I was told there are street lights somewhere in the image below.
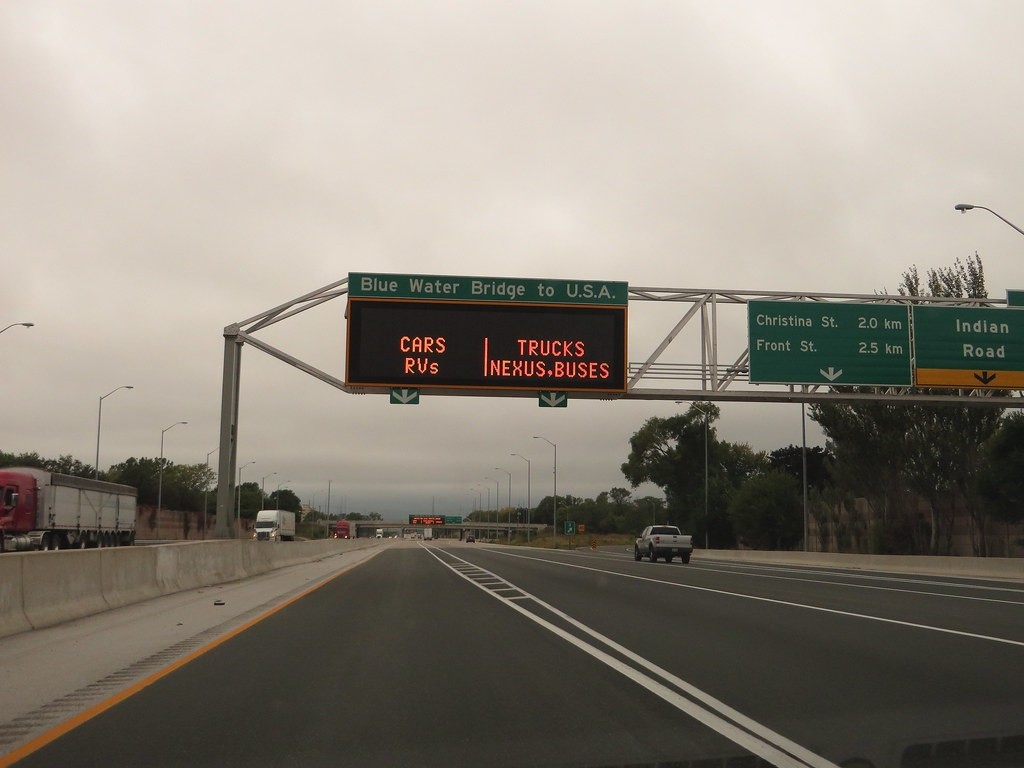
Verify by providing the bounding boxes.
[276,480,291,509]
[156,421,189,540]
[237,461,256,536]
[311,489,326,540]
[534,436,557,551]
[484,476,499,539]
[674,401,709,550]
[94,385,134,482]
[464,482,490,542]
[494,467,511,545]
[511,453,532,543]
[261,472,277,510]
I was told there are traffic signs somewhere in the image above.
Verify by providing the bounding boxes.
[911,303,1024,392]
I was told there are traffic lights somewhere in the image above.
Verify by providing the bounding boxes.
[746,298,914,389]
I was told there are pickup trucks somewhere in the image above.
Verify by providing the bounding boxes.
[634,524,693,565]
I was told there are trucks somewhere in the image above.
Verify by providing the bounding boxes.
[333,520,350,539]
[424,529,432,541]
[375,528,383,539]
[252,509,296,542]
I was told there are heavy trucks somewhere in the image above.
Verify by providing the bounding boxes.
[0,466,140,553]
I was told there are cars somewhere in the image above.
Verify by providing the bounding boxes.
[466,535,476,543]
